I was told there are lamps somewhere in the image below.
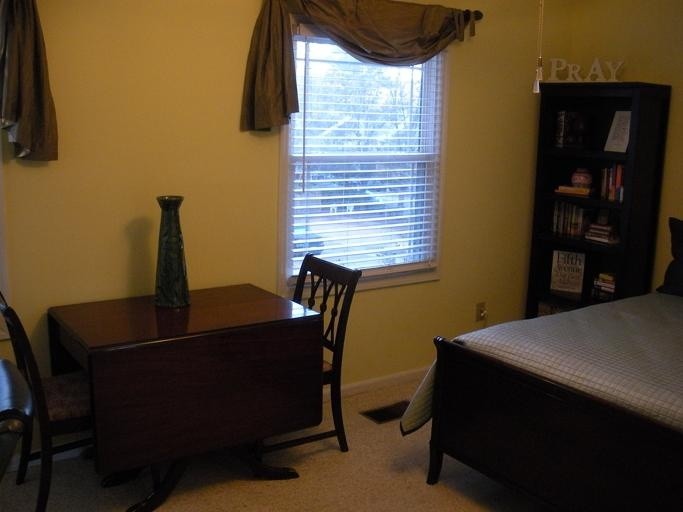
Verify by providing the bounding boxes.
[532,0,546,94]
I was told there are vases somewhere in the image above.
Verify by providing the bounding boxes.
[151,196,189,310]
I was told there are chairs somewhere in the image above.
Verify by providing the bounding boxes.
[1,359,34,479]
[265,253,362,454]
[1,293,93,512]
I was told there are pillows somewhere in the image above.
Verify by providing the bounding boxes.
[656,216,683,295]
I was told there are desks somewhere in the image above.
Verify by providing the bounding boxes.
[49,285,323,512]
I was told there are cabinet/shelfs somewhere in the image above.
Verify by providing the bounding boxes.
[524,81,670,319]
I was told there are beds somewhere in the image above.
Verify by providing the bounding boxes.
[399,293,683,512]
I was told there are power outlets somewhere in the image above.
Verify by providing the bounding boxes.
[476,302,487,320]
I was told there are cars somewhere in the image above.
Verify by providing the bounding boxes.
[288,220,324,262]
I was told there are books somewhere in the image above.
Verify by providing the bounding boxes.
[536,162,624,315]
[552,111,587,150]
[601,109,633,153]
